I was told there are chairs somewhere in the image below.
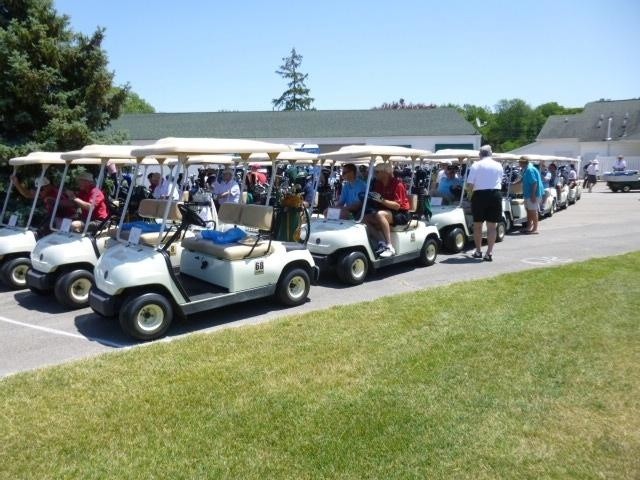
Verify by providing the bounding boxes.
[297,191,319,222]
[84,200,119,236]
[181,202,274,261]
[178,190,189,205]
[393,193,419,231]
[507,181,527,219]
[108,198,184,247]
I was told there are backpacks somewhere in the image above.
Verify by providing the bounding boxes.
[419,189,432,219]
[267,194,311,241]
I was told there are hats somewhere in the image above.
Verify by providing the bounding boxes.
[517,155,528,162]
[78,172,94,183]
[373,163,394,174]
[33,175,54,188]
[222,168,234,175]
[592,160,598,164]
[480,144,492,156]
[617,155,624,158]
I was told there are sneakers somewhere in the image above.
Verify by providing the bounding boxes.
[483,254,493,261]
[526,230,542,234]
[520,229,532,233]
[472,252,483,258]
[374,244,386,255]
[379,248,396,258]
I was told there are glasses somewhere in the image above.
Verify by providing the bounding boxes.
[343,171,349,175]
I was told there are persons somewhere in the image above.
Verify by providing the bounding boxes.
[2,156,585,234]
[366,163,410,258]
[583,161,592,189]
[612,155,626,172]
[586,160,599,193]
[467,144,504,261]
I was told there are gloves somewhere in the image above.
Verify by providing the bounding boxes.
[371,192,384,204]
